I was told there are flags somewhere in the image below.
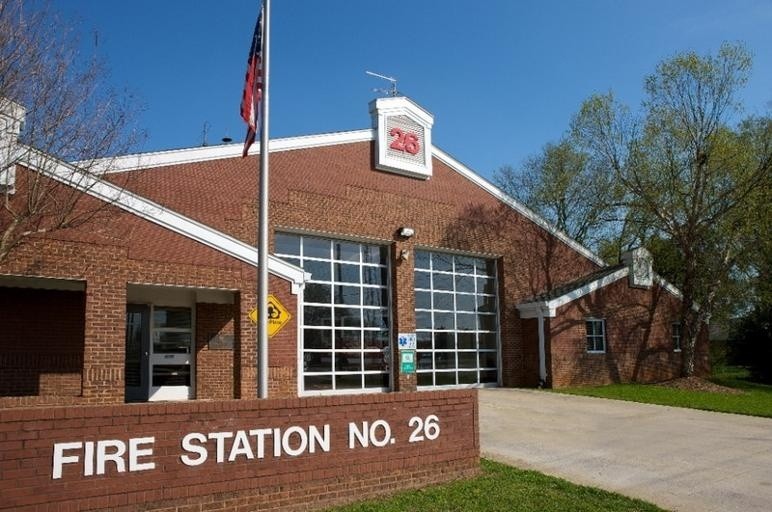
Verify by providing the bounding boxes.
[241,1,266,157]
